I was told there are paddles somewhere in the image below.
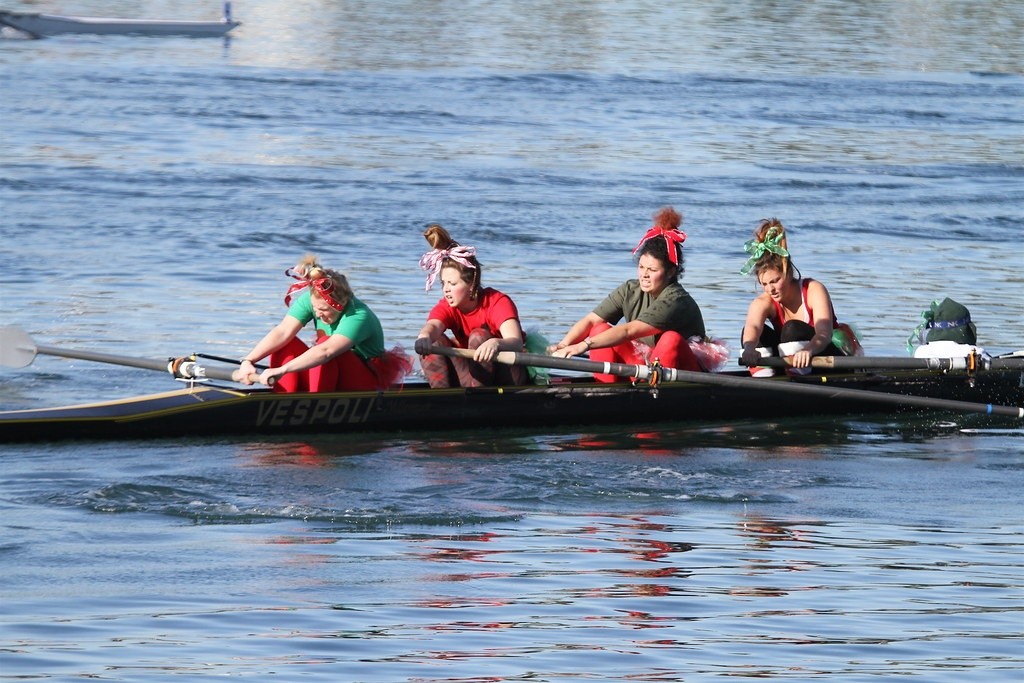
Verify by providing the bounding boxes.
[430,346,1024,418]
[735,353,1024,374]
[0,327,277,387]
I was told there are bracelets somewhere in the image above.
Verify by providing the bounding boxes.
[241,359,254,363]
[585,338,593,350]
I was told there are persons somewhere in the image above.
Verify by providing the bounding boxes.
[550,208,731,385]
[911,296,994,361]
[741,217,863,377]
[238,254,414,393]
[415,225,554,390]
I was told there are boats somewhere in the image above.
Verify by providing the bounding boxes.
[0,350,1024,437]
